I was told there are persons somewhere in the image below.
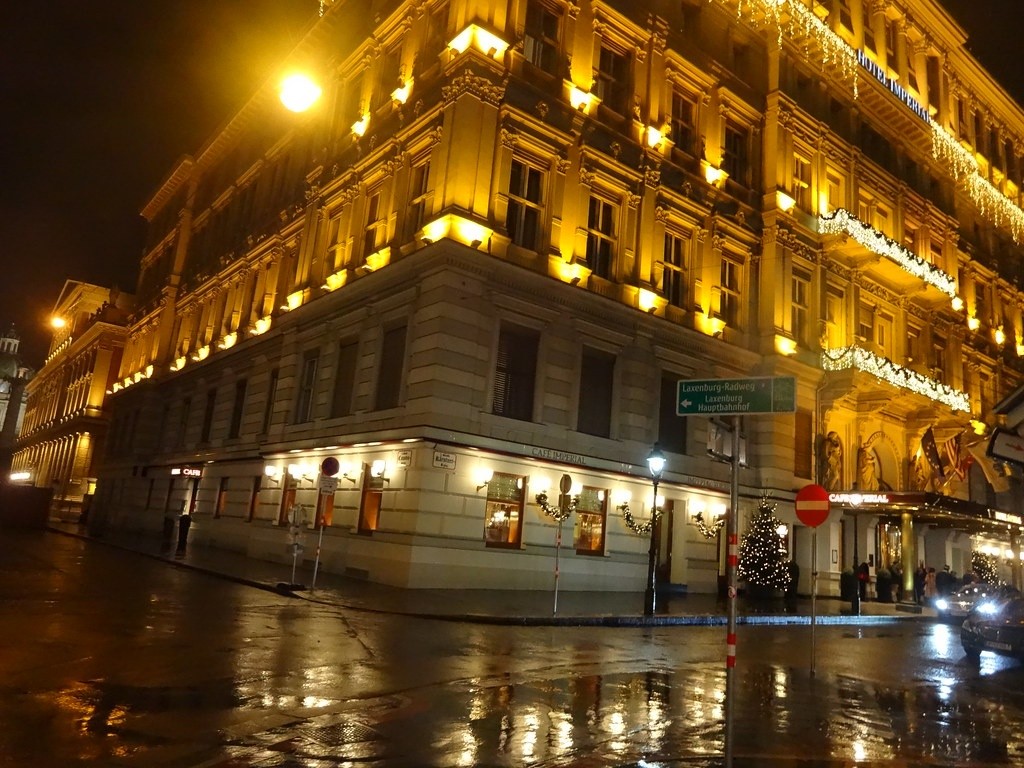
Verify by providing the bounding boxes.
[913,564,973,605]
[821,431,842,492]
[909,455,924,492]
[857,442,879,491]
[840,558,903,604]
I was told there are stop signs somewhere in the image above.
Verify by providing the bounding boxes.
[795,484,830,527]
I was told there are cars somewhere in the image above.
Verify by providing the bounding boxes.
[938,584,993,619]
[960,595,1024,658]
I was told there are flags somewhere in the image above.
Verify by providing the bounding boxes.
[964,434,1010,492]
[943,432,966,482]
[921,428,945,477]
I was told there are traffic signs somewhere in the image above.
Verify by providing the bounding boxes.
[675,376,796,415]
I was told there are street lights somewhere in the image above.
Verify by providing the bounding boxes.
[847,482,863,639]
[644,442,666,616]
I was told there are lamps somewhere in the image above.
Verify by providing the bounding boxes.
[450,47,460,57]
[340,461,356,484]
[652,142,662,152]
[711,179,720,187]
[393,99,402,106]
[785,207,794,214]
[570,277,581,285]
[713,330,722,337]
[280,306,289,312]
[487,46,497,59]
[420,238,432,245]
[470,239,482,249]
[265,465,279,484]
[578,102,587,112]
[371,460,389,484]
[362,264,372,274]
[648,306,657,314]
